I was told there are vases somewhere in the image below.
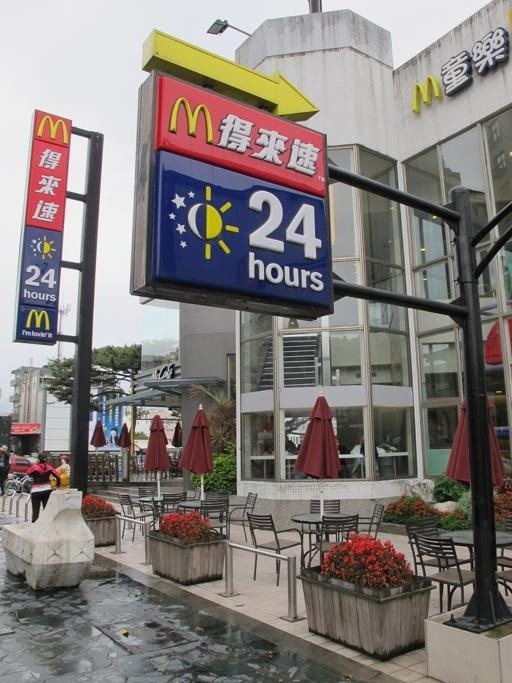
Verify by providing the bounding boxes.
[83,516,117,547]
[297,566,437,662]
[145,530,227,587]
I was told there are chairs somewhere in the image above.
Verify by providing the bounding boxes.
[195,487,201,500]
[342,504,385,542]
[152,497,173,531]
[201,499,229,536]
[320,514,359,566]
[118,492,154,542]
[206,491,229,508]
[496,570,512,597]
[228,492,258,546]
[138,487,160,531]
[310,500,340,515]
[246,512,303,587]
[404,517,470,577]
[163,491,187,508]
[412,531,476,611]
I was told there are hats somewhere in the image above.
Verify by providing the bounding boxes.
[59,454,70,464]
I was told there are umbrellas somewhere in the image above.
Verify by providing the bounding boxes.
[172,421,182,452]
[90,421,106,455]
[445,397,505,486]
[145,415,173,497]
[179,404,214,500]
[117,423,131,448]
[292,393,339,521]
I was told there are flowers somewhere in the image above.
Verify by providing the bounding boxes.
[319,533,415,589]
[158,510,210,542]
[82,495,121,519]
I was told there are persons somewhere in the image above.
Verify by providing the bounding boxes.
[263,435,296,464]
[49,456,70,489]
[27,453,61,523]
[350,435,386,479]
[335,437,350,479]
[0,445,10,495]
[9,450,15,456]
[31,447,41,453]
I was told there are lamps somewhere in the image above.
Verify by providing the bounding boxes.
[207,18,250,37]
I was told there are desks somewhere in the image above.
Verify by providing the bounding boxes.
[291,514,355,580]
[438,531,512,593]
